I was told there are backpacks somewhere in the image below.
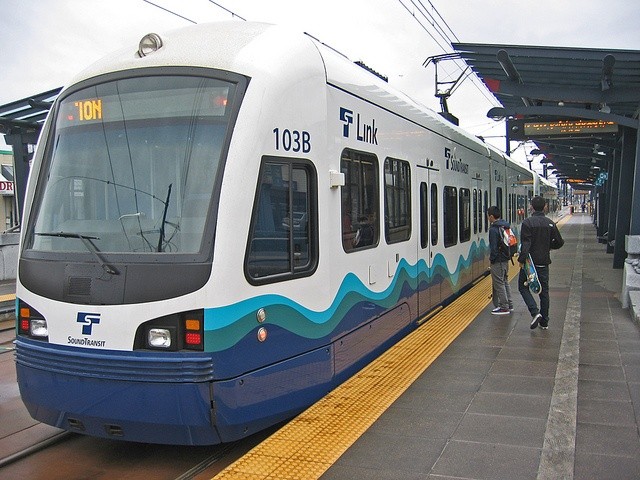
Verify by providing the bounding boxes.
[497,225,517,260]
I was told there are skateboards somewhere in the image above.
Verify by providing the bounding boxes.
[514,243,542,295]
[488,259,497,308]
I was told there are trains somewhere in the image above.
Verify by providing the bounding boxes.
[13,21,563,449]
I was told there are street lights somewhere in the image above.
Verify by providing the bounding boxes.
[529,147,607,160]
[486,104,639,131]
[547,165,598,184]
[539,157,600,167]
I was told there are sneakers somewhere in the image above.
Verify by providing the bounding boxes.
[530,313,543,330]
[491,306,510,315]
[509,306,514,312]
[538,323,548,330]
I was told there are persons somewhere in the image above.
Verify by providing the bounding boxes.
[487,206,517,315]
[519,196,564,328]
